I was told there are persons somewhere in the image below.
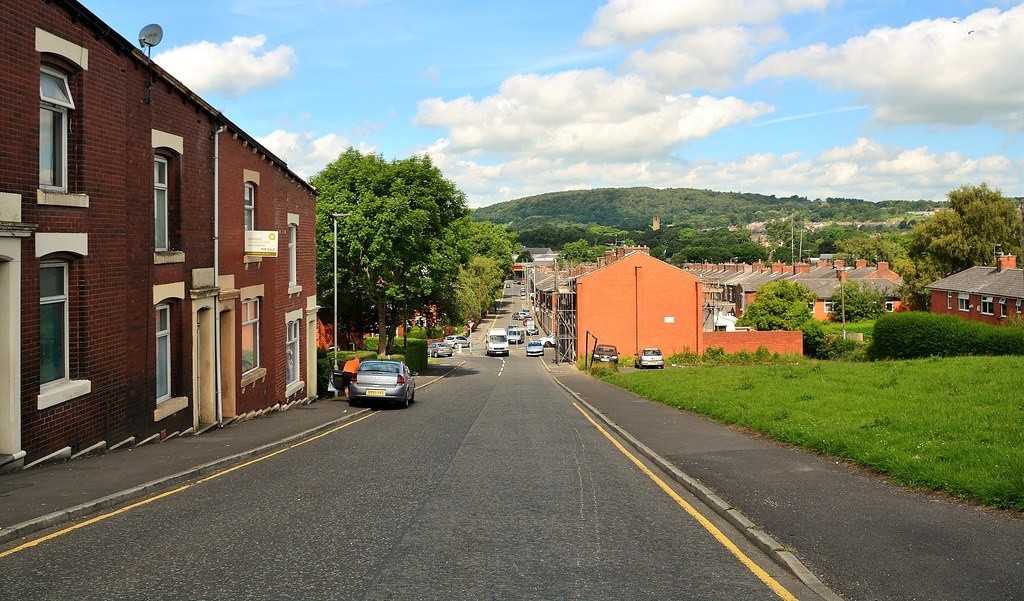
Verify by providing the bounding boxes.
[342,355,361,403]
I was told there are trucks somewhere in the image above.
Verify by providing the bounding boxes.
[485,328,510,357]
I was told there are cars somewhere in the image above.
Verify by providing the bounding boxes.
[427,341,453,357]
[443,335,471,349]
[524,341,545,357]
[591,344,620,364]
[327,358,419,408]
[539,333,556,347]
[634,348,665,369]
[506,280,539,343]
